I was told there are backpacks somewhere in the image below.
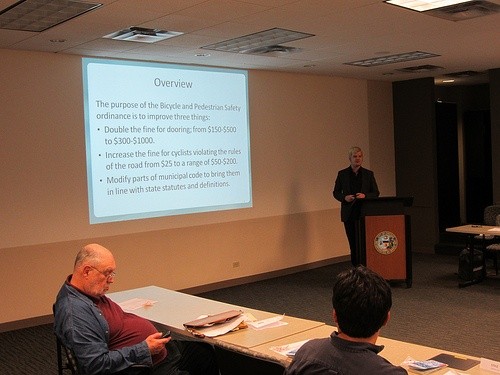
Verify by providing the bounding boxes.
[457,247,486,281]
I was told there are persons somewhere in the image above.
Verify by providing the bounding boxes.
[283,265,409,375]
[53,243,220,375]
[333,147,380,269]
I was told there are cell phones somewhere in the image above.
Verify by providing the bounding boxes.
[162,331,171,338]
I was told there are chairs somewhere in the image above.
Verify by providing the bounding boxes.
[486,214,500,274]
[53,302,85,375]
[467,204,500,241]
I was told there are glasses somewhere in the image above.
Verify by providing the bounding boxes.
[91,266,117,280]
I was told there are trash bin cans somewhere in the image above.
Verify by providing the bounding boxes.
[459,247,484,284]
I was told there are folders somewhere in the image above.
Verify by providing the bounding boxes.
[183,309,244,337]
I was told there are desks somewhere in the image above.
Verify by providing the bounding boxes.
[104,285,500,375]
[448,223,500,278]
